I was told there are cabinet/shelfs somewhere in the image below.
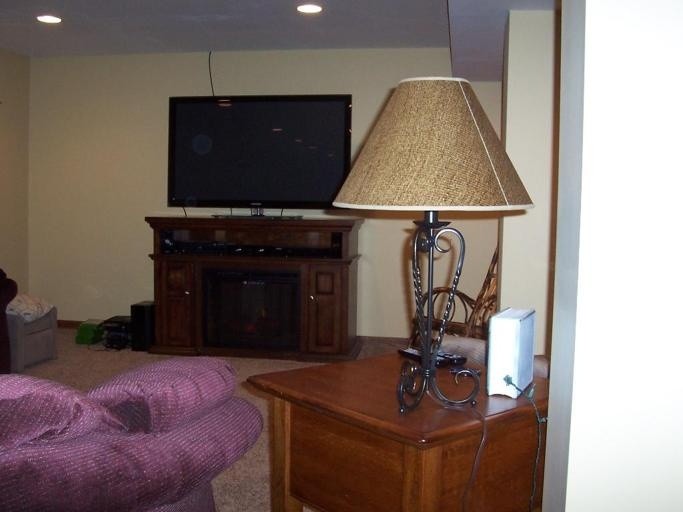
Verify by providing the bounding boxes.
[145,217,365,361]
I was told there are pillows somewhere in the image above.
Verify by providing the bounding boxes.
[0,373,130,454]
[84,357,238,436]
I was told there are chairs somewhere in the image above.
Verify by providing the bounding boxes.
[408,243,498,350]
[5,305,58,372]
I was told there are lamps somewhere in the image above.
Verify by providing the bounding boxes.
[331,76,534,416]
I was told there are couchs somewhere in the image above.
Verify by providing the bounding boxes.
[0,355,263,512]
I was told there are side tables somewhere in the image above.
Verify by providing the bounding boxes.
[247,350,549,512]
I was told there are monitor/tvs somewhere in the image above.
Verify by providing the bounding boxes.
[167,94,352,220]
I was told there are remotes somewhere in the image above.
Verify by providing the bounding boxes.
[398,347,450,367]
[410,344,467,366]
